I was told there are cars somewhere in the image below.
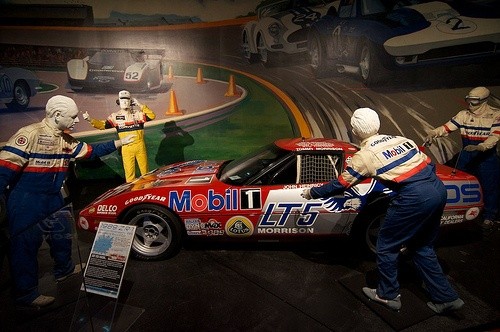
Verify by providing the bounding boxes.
[77,138,484,263]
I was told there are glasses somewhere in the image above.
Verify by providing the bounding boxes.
[465,96,489,105]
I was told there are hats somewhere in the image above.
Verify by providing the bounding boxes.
[118,91,131,99]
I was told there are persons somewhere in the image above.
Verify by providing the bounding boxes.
[301,107,464,313]
[0,95,136,305]
[82,90,156,182]
[427,86,500,237]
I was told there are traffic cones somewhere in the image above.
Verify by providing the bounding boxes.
[165,91,184,116]
[223,74,243,98]
[195,66,207,84]
[166,66,176,80]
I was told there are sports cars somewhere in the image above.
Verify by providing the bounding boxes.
[241,0,340,68]
[306,0,500,87]
[64,47,166,93]
[0,66,41,111]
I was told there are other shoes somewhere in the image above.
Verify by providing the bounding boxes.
[480,220,494,228]
[32,295,55,307]
[427,298,465,314]
[363,287,400,309]
[57,262,87,280]
